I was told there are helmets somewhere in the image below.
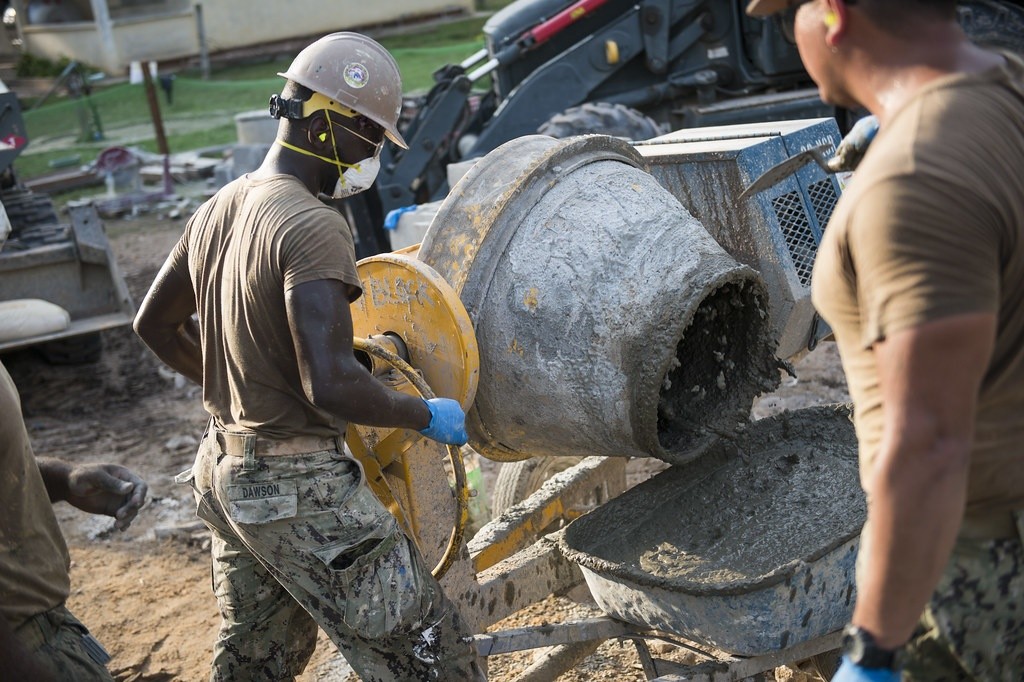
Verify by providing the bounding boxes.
[277,31,410,152]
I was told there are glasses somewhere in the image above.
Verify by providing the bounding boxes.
[320,115,387,160]
[774,0,859,47]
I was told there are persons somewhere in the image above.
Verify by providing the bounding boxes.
[132,31,486,681]
[0,358,149,682]
[744,0,1024,682]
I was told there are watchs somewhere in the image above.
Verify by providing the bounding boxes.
[841,623,905,668]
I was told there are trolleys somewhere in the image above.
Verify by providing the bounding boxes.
[462,400,870,681]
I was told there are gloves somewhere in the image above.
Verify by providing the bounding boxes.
[383,202,418,228]
[419,397,468,447]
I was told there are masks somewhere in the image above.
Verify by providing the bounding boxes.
[332,138,382,199]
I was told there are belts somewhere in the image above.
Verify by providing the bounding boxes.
[956,503,1024,542]
[205,417,337,466]
